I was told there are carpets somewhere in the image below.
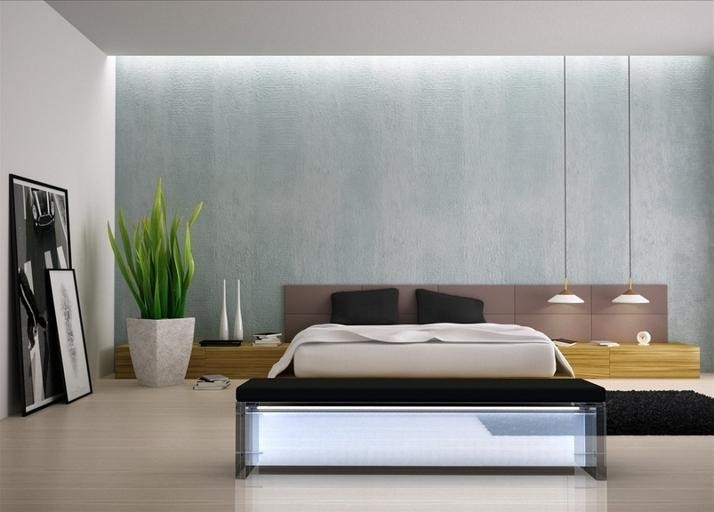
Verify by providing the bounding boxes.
[602,389,713,438]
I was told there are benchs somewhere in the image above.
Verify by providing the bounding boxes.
[231,377,611,483]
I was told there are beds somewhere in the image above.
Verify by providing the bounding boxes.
[289,320,560,378]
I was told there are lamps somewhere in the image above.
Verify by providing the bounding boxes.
[546,56,652,305]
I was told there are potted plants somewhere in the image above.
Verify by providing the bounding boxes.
[107,172,207,387]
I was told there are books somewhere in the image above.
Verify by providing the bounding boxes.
[604,343,619,347]
[590,339,617,345]
[252,332,284,348]
[551,337,576,346]
[192,374,231,391]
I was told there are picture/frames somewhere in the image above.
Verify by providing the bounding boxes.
[10,172,94,417]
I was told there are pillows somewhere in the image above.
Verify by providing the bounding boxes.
[329,287,487,324]
